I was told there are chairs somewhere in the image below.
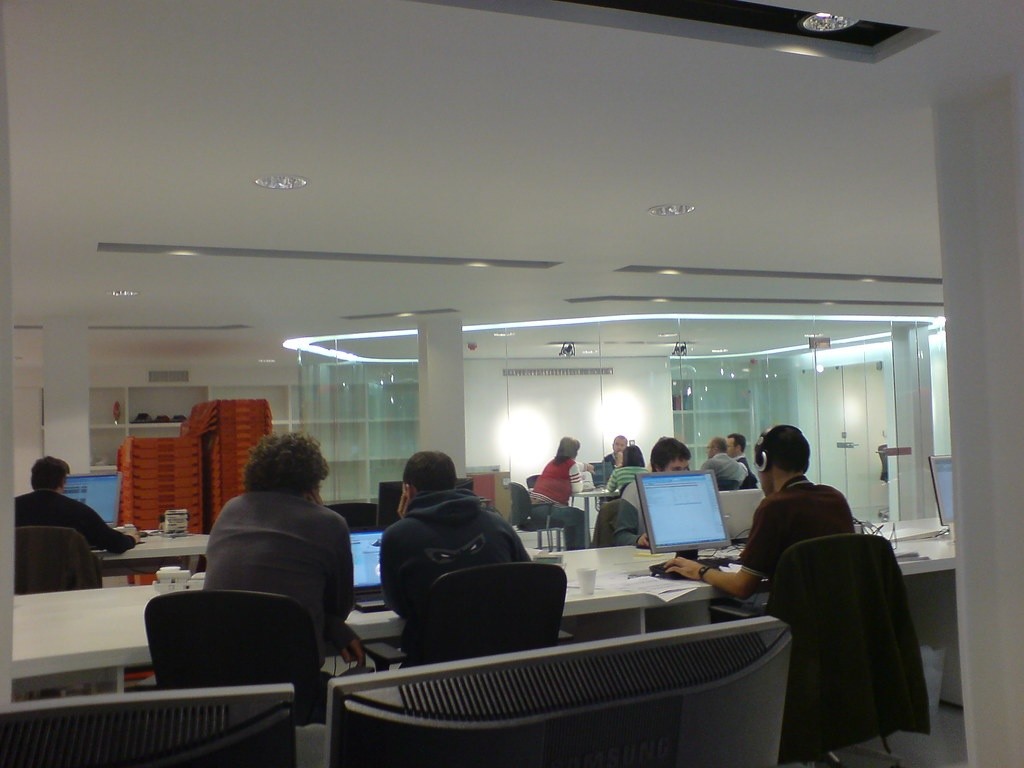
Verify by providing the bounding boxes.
[510,482,566,554]
[15,526,102,595]
[145,593,319,727]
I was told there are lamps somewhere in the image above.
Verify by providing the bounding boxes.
[671,341,687,356]
[559,341,575,357]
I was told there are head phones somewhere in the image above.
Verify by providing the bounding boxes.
[753,424,801,472]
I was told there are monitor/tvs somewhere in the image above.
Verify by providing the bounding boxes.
[634,469,733,560]
[61,471,123,528]
[928,454,955,526]
[0,682,299,768]
[326,614,792,768]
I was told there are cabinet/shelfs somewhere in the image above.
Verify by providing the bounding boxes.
[89,383,212,467]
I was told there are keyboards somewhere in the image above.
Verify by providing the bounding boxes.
[649,557,744,580]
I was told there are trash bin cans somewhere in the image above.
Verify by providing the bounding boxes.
[918,643,947,717]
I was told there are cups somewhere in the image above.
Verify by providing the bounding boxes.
[576,568,597,595]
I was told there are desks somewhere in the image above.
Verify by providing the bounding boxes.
[0,490,961,713]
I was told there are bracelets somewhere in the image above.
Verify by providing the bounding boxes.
[699,565,721,582]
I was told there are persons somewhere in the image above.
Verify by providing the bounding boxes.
[613,436,691,547]
[700,437,748,488]
[602,436,628,469]
[528,437,595,550]
[14,456,140,554]
[379,451,532,655]
[727,433,753,489]
[203,429,367,726]
[606,445,649,496]
[664,421,855,599]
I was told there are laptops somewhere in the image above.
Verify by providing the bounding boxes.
[589,462,614,488]
[375,481,405,529]
[348,525,393,613]
